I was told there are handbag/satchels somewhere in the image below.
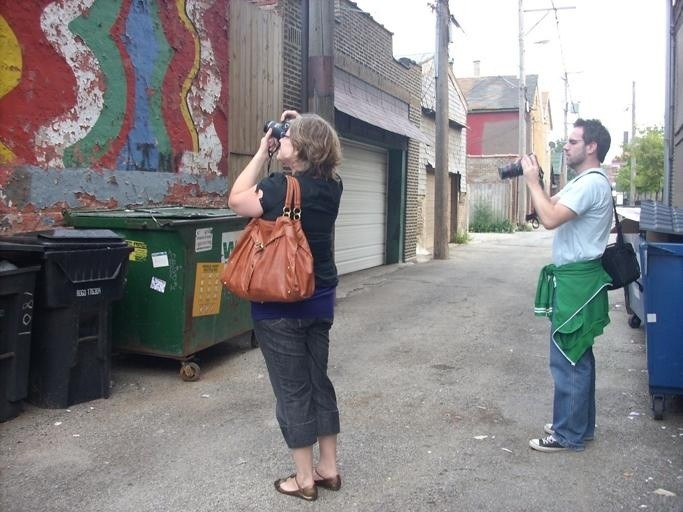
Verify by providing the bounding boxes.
[602,242,641,292]
[217,176,316,305]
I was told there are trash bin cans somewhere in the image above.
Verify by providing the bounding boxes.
[0,229,135,424]
[618,233,646,328]
[62,207,259,382]
[638,241,683,421]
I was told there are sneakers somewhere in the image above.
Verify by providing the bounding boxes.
[528,435,566,453]
[544,422,594,441]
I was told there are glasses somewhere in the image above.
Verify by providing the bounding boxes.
[280,132,289,139]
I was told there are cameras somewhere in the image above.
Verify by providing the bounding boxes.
[263,118,293,141]
[498,152,544,180]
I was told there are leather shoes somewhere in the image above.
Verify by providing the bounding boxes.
[273,475,317,501]
[288,467,341,491]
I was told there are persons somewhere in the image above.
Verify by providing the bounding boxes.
[226,106,344,499]
[514,118,615,450]
